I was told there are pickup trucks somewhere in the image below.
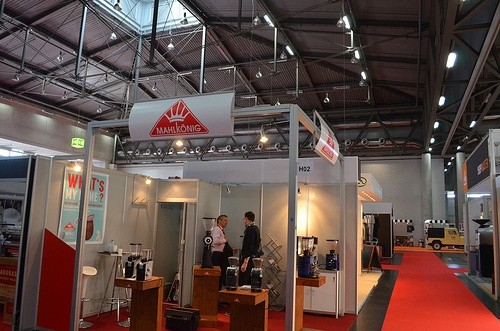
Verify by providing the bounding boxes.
[425,228,464,250]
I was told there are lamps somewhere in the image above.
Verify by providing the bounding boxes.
[180,11,189,25]
[256,66,263,79]
[62,91,69,101]
[280,45,288,60]
[337,3,368,87]
[113,0,121,9]
[56,51,64,63]
[167,38,175,49]
[226,187,231,194]
[259,124,272,143]
[152,81,159,91]
[323,91,330,103]
[252,11,263,25]
[109,18,117,38]
[97,105,103,112]
[12,74,20,82]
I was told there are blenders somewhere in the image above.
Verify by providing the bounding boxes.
[225,257,239,291]
[297,238,318,278]
[249,258,263,292]
[325,239,339,271]
[124,242,153,282]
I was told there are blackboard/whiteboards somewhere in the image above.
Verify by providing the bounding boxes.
[363,245,380,268]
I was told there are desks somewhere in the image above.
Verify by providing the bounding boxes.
[191,264,221,322]
[295,276,326,330]
[217,285,269,331]
[114,277,163,331]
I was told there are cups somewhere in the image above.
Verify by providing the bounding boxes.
[112,244,117,252]
[118,249,123,254]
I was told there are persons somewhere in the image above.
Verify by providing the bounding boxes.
[238,212,261,286]
[211,215,229,266]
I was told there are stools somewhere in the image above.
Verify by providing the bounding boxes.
[78,265,97,329]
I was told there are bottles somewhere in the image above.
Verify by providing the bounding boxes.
[109,240,115,252]
